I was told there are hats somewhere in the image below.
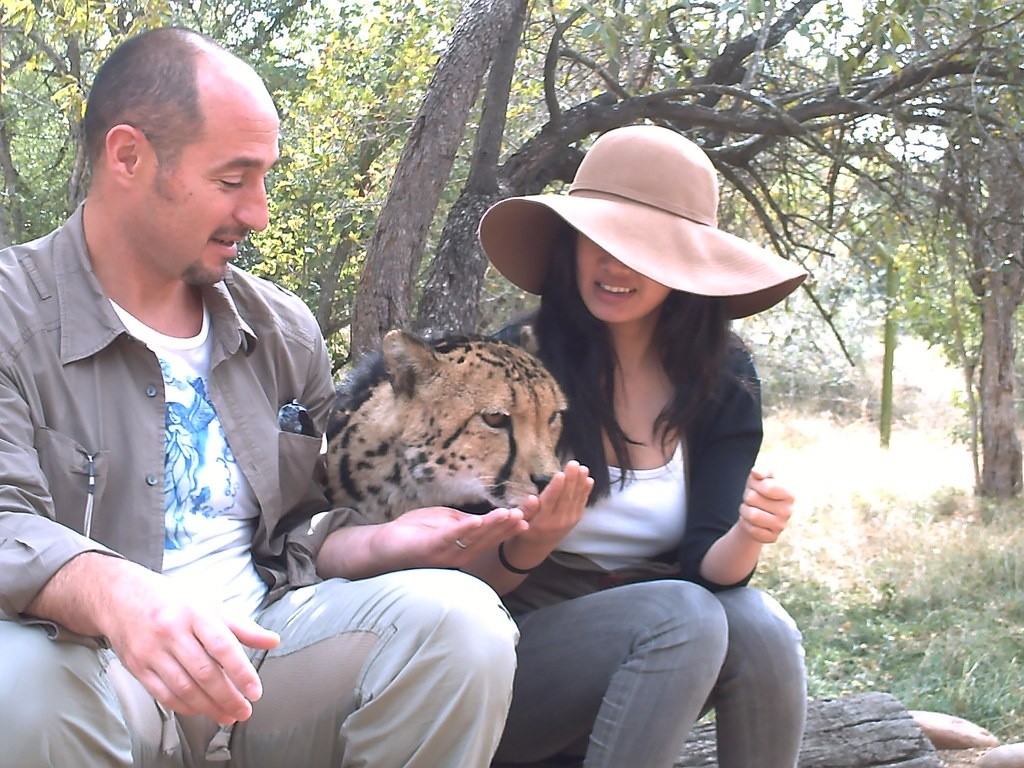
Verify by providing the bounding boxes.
[477,125,808,320]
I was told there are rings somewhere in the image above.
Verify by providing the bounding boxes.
[455,539,467,549]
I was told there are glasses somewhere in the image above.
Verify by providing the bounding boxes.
[278,398,315,437]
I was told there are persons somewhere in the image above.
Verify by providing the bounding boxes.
[478,126,810,768]
[0,27,530,768]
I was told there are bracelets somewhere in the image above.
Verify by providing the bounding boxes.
[498,541,548,574]
